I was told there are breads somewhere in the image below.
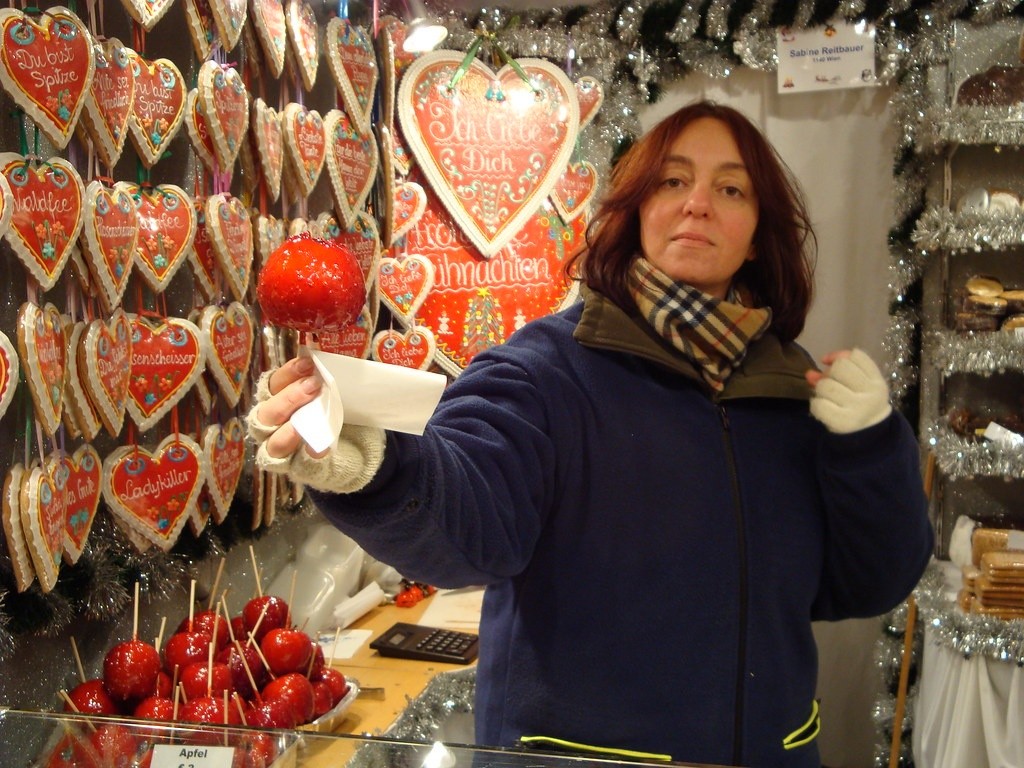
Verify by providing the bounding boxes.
[971,528,1024,567]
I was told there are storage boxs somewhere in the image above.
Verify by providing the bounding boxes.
[0,706,724,768]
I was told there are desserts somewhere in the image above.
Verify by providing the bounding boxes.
[47,596,346,767]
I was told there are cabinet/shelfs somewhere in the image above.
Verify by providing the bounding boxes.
[910,25,1024,562]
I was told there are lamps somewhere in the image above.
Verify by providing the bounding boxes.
[399,0,447,54]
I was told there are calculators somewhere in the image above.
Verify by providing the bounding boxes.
[370,622,479,665]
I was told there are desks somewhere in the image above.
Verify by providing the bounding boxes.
[286,585,490,768]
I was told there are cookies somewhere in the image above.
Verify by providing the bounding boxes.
[959,550,1024,621]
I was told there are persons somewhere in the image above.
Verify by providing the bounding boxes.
[248,101,937,768]
